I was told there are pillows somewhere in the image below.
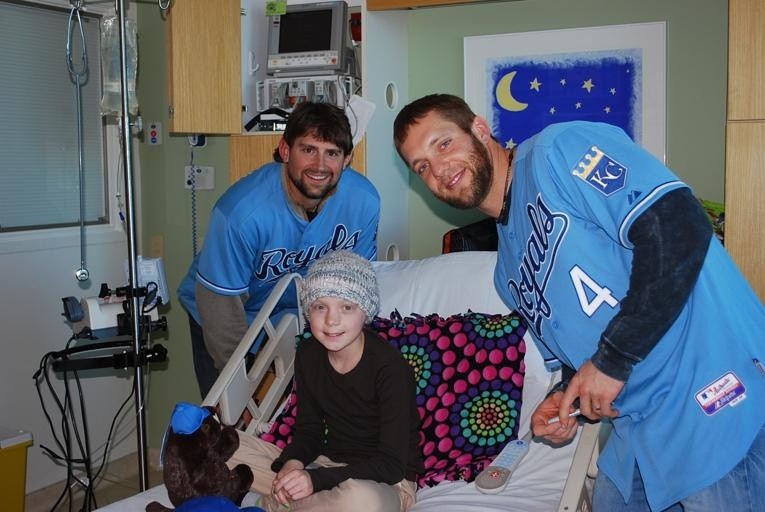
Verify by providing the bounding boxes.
[260,312,527,485]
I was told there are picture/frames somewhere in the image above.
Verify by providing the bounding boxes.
[462,21,668,172]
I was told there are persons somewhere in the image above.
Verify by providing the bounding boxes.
[240,248,421,512]
[392,93,765,512]
[176,100,381,431]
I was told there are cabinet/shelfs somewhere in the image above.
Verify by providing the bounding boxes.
[167,0,365,187]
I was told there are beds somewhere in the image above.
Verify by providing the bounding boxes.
[88,251,601,512]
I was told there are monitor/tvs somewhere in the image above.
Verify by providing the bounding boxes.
[266,1,350,74]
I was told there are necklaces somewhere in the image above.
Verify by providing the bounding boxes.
[496,151,513,224]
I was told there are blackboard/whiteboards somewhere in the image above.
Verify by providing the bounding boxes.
[0,0,110,233]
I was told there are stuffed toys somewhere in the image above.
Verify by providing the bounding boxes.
[144,401,256,511]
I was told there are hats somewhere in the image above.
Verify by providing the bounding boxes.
[298,249,382,324]
